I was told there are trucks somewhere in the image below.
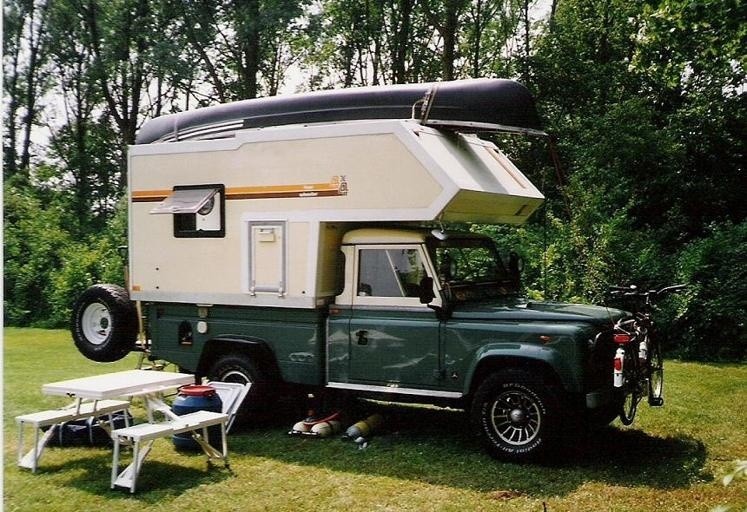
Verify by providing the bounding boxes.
[123,128,636,463]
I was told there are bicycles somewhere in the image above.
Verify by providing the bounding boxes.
[608,281,692,433]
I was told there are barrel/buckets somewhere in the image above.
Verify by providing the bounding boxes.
[172,384,222,450]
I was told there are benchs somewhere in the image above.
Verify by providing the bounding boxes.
[110,407,228,493]
[16,397,126,475]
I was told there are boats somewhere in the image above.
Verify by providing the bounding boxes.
[126,72,549,142]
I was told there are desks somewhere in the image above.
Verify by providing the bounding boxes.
[41,368,196,423]
